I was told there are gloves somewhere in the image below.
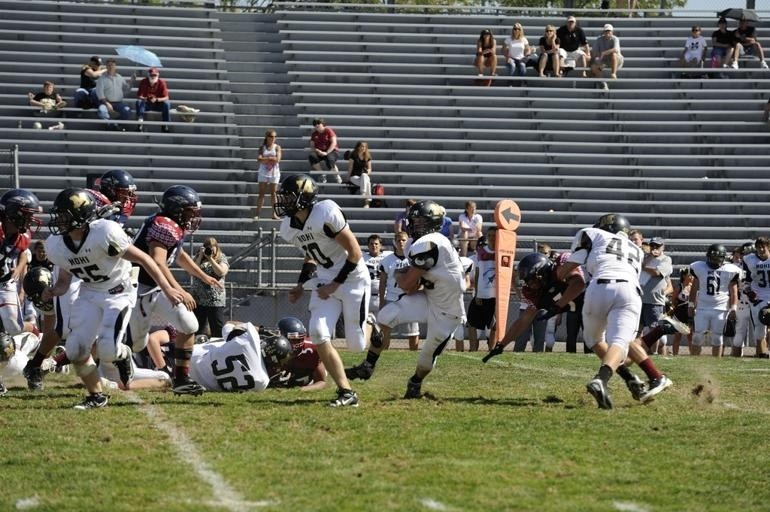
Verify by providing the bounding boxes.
[266,370,292,388]
[482,341,504,363]
[529,303,562,325]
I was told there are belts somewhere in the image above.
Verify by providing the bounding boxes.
[597,279,641,295]
[371,294,378,296]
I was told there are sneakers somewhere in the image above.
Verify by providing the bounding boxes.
[600,82,608,90]
[327,316,422,409]
[585,374,674,410]
[321,175,328,183]
[730,62,739,69]
[657,313,691,336]
[760,62,768,68]
[172,374,206,397]
[22,346,72,396]
[333,173,342,184]
[112,344,135,390]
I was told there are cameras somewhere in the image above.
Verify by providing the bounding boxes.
[203,247,214,256]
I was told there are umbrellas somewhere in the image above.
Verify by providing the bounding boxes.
[115,45,166,74]
[718,8,760,21]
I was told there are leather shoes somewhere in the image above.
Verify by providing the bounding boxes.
[105,118,171,133]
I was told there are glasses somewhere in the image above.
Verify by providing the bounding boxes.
[482,27,554,36]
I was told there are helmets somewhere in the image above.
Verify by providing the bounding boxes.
[161,185,203,234]
[0,170,138,235]
[259,317,307,368]
[405,200,446,238]
[476,235,489,252]
[22,266,53,311]
[598,214,631,239]
[273,174,319,217]
[0,331,16,362]
[517,253,556,305]
[705,244,726,269]
[760,305,770,326]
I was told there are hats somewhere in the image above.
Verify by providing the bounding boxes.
[90,56,103,65]
[567,15,577,23]
[648,236,664,246]
[603,23,613,32]
[148,68,160,76]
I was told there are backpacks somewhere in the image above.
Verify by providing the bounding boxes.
[370,182,388,208]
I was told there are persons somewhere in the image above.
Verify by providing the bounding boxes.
[0,169,498,409]
[482,213,769,408]
[135,68,173,134]
[472,15,624,90]
[308,117,343,185]
[74,56,107,118]
[679,16,768,70]
[95,58,137,132]
[347,141,373,208]
[28,80,64,131]
[253,130,282,220]
